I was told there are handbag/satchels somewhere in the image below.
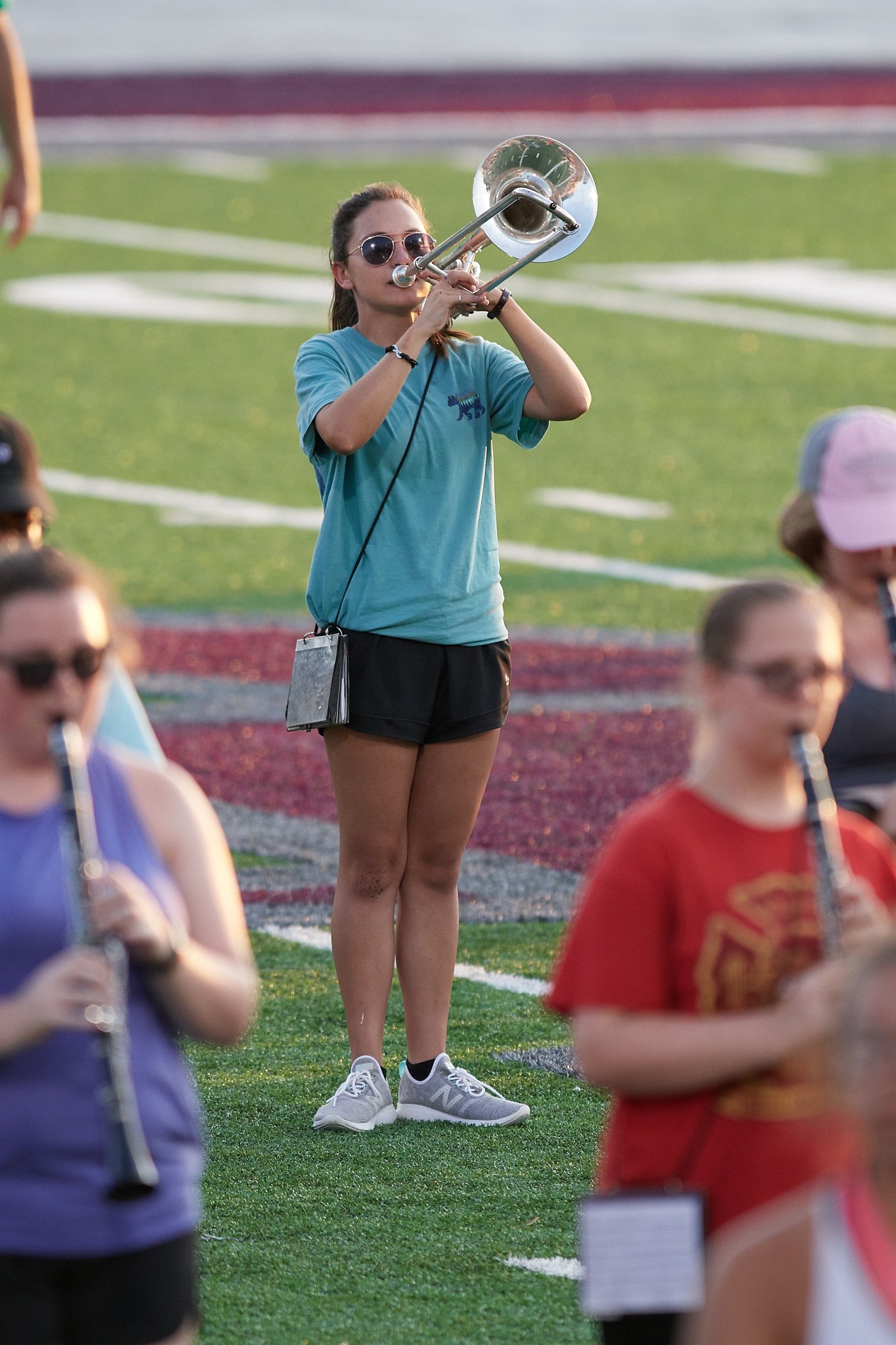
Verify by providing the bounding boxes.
[285,623,351,731]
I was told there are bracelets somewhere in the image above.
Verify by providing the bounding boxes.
[384,344,419,369]
[487,287,513,320]
[131,945,179,977]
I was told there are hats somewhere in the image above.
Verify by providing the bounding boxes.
[1,408,56,522]
[800,407,896,550]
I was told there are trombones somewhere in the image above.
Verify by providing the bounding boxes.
[392,134,599,320]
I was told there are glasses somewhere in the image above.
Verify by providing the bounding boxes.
[7,639,115,691]
[344,232,436,268]
[733,660,853,706]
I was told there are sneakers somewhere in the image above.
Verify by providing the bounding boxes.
[311,1055,396,1132]
[396,1052,531,1128]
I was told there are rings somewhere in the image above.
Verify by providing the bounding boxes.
[458,295,462,303]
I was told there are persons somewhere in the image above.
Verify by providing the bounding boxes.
[0,0,43,256]
[776,404,896,858]
[572,578,896,1342]
[1,548,259,1345]
[0,409,168,771]
[291,182,593,1129]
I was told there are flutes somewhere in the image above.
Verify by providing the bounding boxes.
[874,569,896,666]
[788,730,877,1085]
[50,712,164,1209]
